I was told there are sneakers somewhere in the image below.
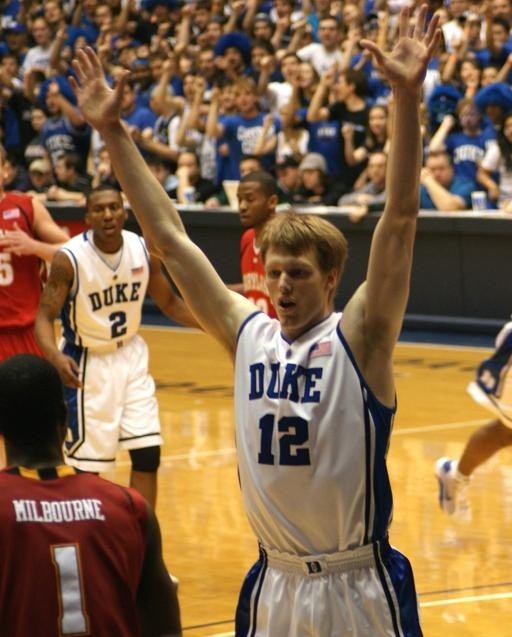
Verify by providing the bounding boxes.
[434,457,463,515]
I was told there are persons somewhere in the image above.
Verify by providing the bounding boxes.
[431,320,512,516]
[1,144,73,357]
[0,350,182,636]
[222,169,280,322]
[68,1,442,636]
[33,184,206,598]
[0,0,512,223]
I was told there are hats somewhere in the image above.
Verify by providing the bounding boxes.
[299,154,326,171]
[30,160,47,174]
